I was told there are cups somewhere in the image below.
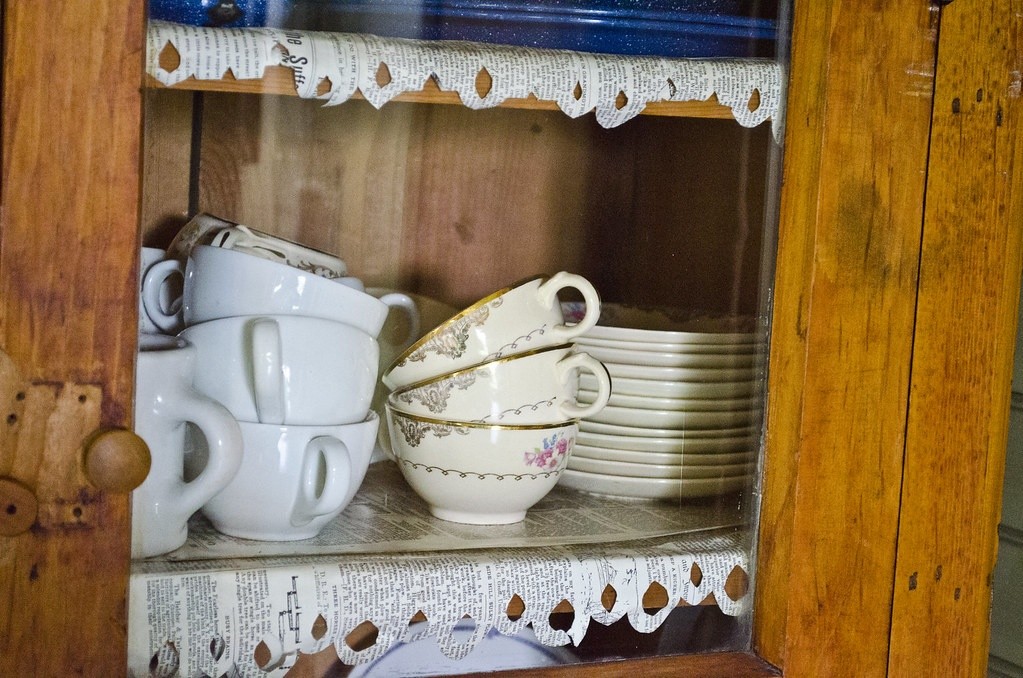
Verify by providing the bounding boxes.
[130,247,421,561]
[163,212,347,278]
[379,271,612,526]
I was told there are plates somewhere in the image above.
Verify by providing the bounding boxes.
[556,299,768,501]
[322,0,781,58]
[344,618,584,678]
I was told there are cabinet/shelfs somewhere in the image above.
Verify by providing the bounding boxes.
[0,0,1021,677]
